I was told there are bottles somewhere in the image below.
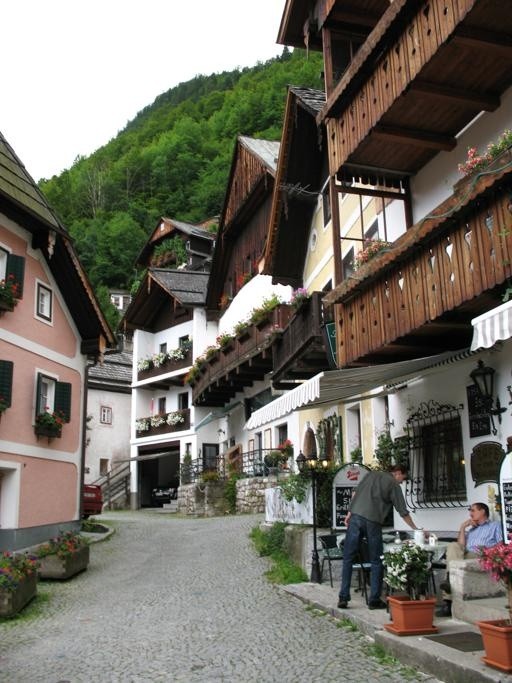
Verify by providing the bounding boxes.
[394,530,401,545]
[405,540,413,548]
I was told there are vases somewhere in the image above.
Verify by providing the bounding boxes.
[476,621,511,672]
[281,463,287,469]
[0,294,15,310]
[34,426,61,437]
[384,594,439,636]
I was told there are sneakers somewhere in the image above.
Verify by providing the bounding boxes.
[337,598,348,608]
[367,599,387,610]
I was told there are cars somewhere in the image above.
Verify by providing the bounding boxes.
[151,480,180,507]
[81,483,105,521]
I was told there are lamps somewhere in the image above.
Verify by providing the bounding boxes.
[470,358,508,434]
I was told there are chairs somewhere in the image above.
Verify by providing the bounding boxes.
[317,530,459,604]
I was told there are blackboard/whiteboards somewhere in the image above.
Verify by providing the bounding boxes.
[332,463,372,532]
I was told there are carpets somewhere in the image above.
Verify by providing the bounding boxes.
[423,630,484,652]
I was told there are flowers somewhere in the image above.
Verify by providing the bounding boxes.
[37,405,65,429]
[349,236,390,270]
[277,439,294,463]
[2,275,22,295]
[136,347,185,372]
[457,128,512,174]
[0,550,41,592]
[472,531,512,620]
[181,286,309,388]
[135,411,185,432]
[39,528,91,561]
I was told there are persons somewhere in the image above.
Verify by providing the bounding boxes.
[431,502,504,616]
[336,461,431,611]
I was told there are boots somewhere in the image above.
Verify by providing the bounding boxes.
[434,598,452,617]
[439,571,452,595]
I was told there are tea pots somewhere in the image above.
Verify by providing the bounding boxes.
[413,529,426,546]
